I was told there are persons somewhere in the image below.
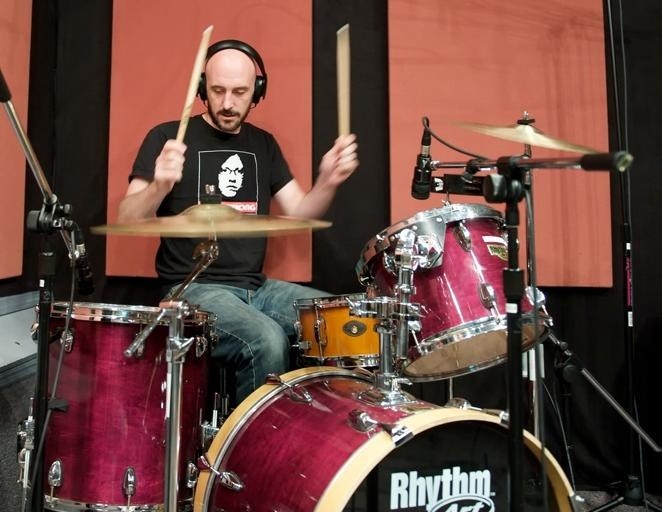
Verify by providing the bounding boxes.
[113,40,361,405]
[207,149,252,202]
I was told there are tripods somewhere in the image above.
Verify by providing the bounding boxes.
[590,1,662,511]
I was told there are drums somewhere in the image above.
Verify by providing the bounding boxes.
[16,301,218,511]
[355,203,554,381]
[292,292,379,369]
[193,365,580,512]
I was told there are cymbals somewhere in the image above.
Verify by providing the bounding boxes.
[90,205,333,238]
[437,117,599,155]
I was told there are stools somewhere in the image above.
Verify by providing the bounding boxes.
[210,357,236,417]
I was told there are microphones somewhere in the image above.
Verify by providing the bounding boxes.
[412,138,432,200]
[74,254,96,303]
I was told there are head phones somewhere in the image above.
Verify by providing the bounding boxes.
[197,40,268,105]
